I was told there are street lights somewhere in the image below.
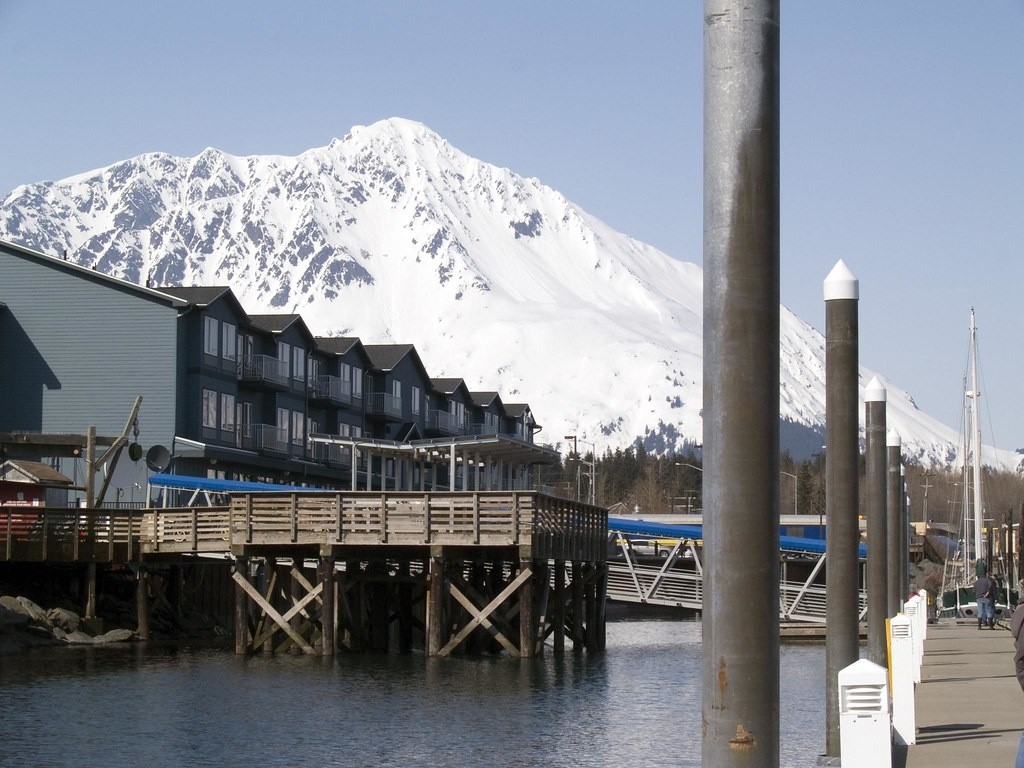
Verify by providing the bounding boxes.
[578,438,596,507]
[811,453,822,541]
[565,434,579,502]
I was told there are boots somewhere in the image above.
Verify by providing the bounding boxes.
[988,618,994,630]
[978,618,982,630]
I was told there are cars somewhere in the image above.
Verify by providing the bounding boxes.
[626,539,671,558]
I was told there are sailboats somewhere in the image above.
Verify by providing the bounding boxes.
[920,305,1018,622]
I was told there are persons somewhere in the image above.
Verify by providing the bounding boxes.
[1010,597,1024,768]
[972,572,998,630]
